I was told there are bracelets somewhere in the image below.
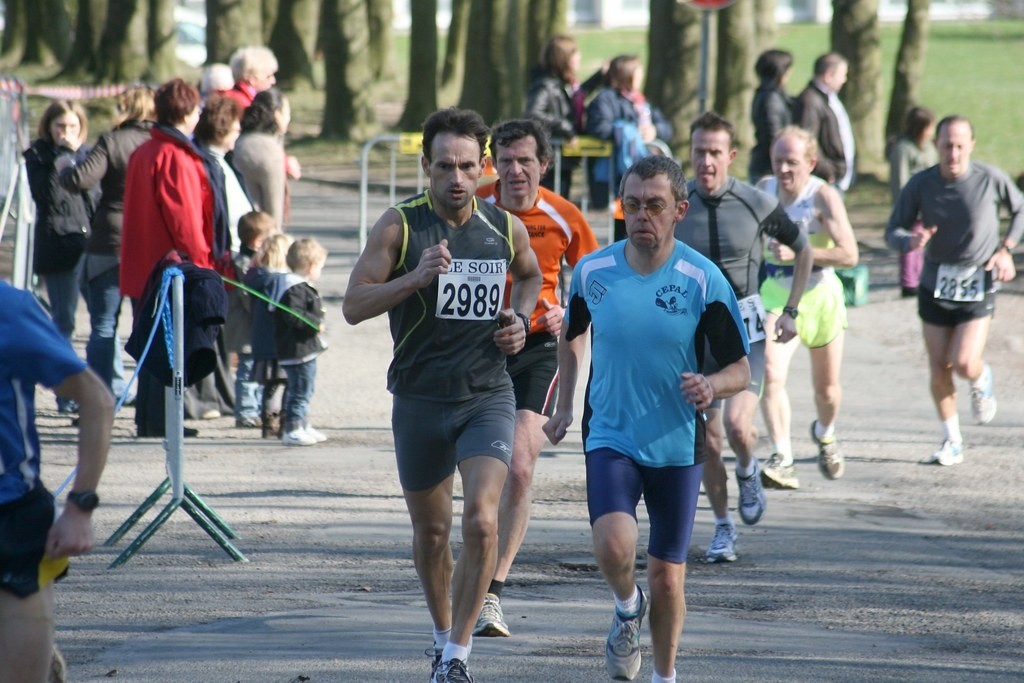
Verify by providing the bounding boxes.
[1001,244,1014,254]
[516,312,531,332]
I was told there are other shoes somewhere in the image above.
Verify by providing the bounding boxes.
[136,423,199,437]
[236,418,263,427]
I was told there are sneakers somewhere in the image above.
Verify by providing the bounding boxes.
[760,452,800,490]
[605,585,647,680]
[281,429,317,445]
[707,521,738,562]
[932,443,966,464]
[811,419,845,480]
[303,426,326,441]
[969,363,997,423]
[431,657,474,683]
[471,591,510,637]
[425,640,444,668]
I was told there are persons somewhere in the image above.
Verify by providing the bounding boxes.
[884,116,1024,465]
[55,46,300,419]
[587,56,673,243]
[673,114,817,563]
[526,36,610,199]
[21,101,136,412]
[755,126,860,485]
[342,110,601,683]
[0,277,114,683]
[225,211,328,445]
[888,107,937,296]
[121,79,212,438]
[746,50,794,187]
[796,52,855,200]
[544,156,751,683]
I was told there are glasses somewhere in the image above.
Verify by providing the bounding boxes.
[619,200,678,217]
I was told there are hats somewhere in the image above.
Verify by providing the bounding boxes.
[200,63,235,93]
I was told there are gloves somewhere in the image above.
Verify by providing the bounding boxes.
[735,456,766,525]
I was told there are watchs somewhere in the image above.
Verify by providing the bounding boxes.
[67,490,99,510]
[782,306,798,318]
